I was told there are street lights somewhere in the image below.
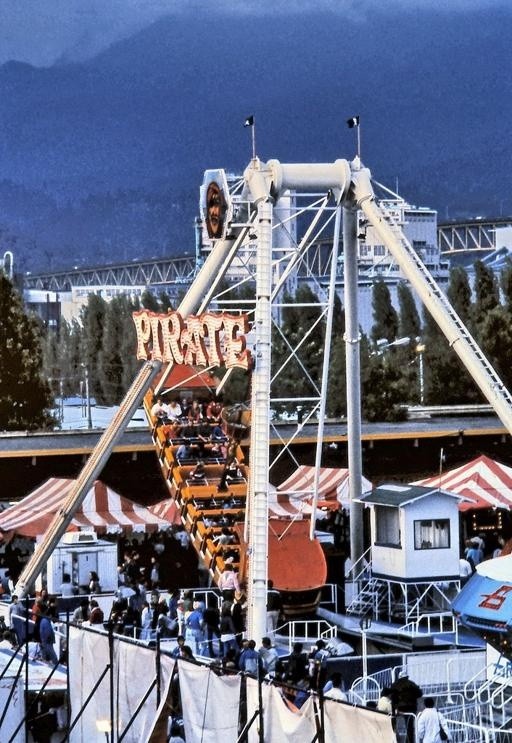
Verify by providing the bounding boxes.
[415,333,427,406]
[371,336,389,349]
[55,359,93,429]
[383,335,411,350]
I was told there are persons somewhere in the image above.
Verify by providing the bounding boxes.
[28,694,64,743]
[1,545,104,664]
[109,525,204,665]
[286,640,348,701]
[167,706,185,743]
[376,672,453,743]
[213,527,238,563]
[203,564,283,675]
[464,531,504,564]
[184,494,246,510]
[150,397,242,484]
[204,510,244,528]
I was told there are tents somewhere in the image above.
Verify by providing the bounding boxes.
[407,455,512,513]
[268,465,372,521]
[1,477,171,537]
[148,498,182,525]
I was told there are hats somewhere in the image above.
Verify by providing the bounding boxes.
[398,671,408,679]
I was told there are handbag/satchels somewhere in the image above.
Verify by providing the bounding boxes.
[440,727,447,740]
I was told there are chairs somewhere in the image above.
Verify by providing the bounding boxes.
[152,395,246,575]
